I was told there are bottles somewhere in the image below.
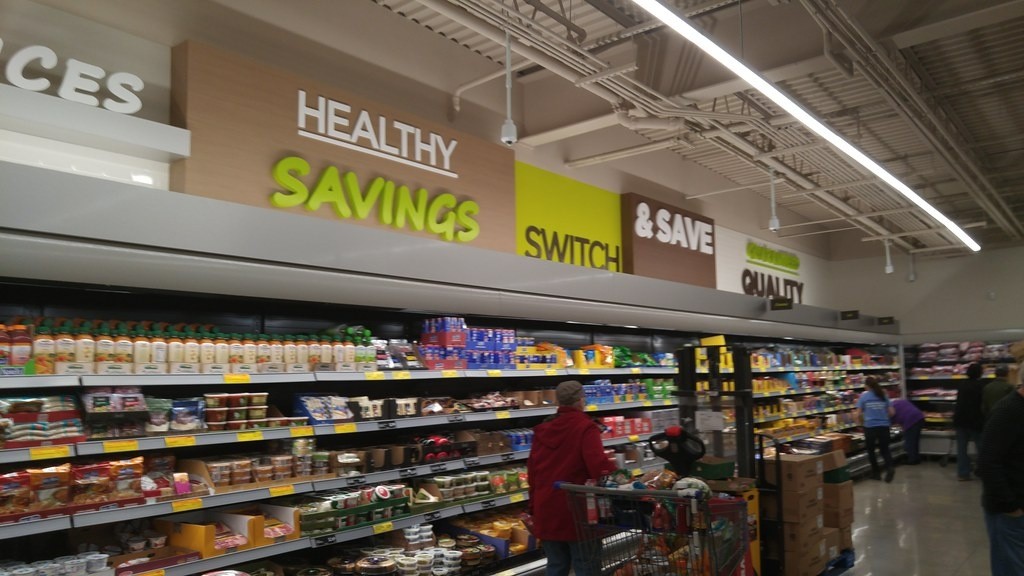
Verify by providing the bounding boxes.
[0,316,536,376]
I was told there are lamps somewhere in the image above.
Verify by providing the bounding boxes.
[619,0,984,253]
[767,174,781,233]
[499,32,518,147]
[907,254,916,282]
[883,240,894,274]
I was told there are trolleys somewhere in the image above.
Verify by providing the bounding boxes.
[940,409,982,477]
[553,479,749,576]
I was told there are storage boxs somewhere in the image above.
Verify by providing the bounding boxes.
[0,331,854,576]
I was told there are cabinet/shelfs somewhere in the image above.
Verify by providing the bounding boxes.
[0,156,908,576]
[903,329,1024,455]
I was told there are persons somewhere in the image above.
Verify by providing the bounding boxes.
[527,381,617,576]
[950,364,986,481]
[889,398,927,465]
[981,364,1016,417]
[854,377,895,483]
[981,364,1024,576]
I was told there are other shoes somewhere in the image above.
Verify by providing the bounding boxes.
[869,474,881,480]
[885,468,896,483]
[957,472,974,481]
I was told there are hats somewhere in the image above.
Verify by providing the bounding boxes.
[995,364,1009,374]
[555,380,585,404]
[1016,367,1024,385]
